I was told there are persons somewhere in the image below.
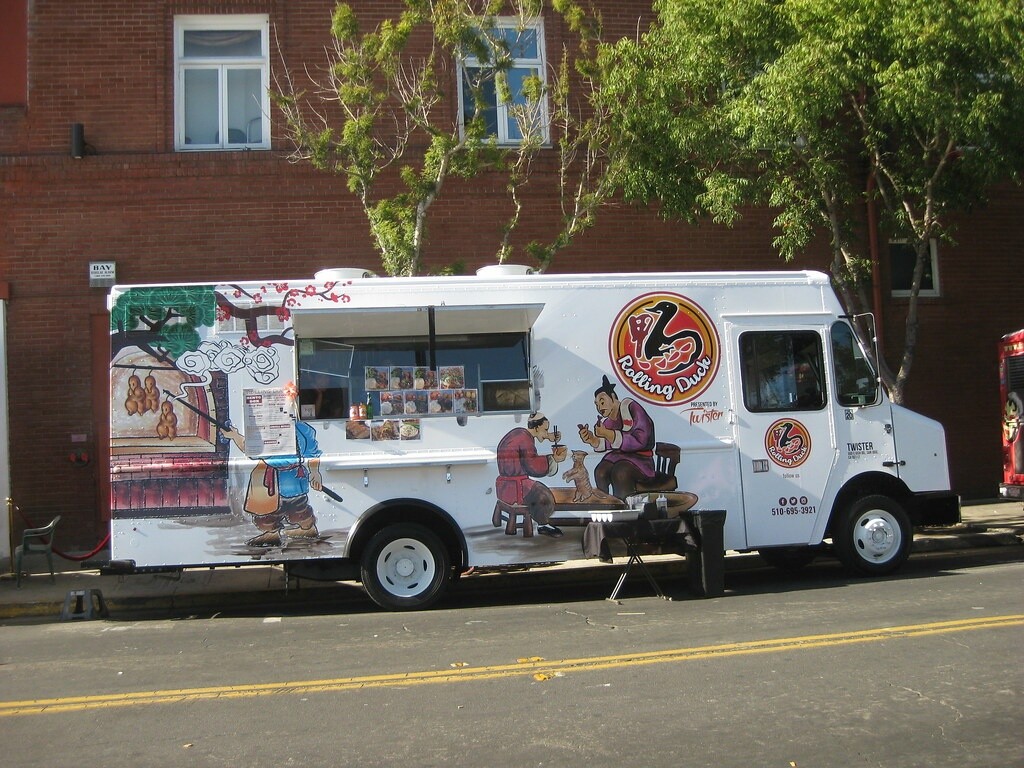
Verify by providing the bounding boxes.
[784,356,822,409]
[300,361,343,419]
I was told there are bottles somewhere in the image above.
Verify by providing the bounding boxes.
[359,400,366,419]
[366,392,374,420]
[349,400,358,419]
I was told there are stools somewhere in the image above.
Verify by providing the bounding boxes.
[60,589,110,623]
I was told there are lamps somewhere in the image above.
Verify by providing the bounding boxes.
[70,124,95,159]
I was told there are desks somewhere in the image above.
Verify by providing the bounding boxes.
[582,519,683,605]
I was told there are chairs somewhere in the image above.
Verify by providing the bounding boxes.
[14,515,61,589]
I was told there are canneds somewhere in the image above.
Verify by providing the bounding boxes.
[358,405,367,419]
[349,405,358,419]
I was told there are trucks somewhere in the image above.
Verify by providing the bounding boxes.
[104,262,964,612]
[994,328,1024,504]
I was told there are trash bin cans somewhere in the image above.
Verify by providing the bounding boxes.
[680,507,728,598]
